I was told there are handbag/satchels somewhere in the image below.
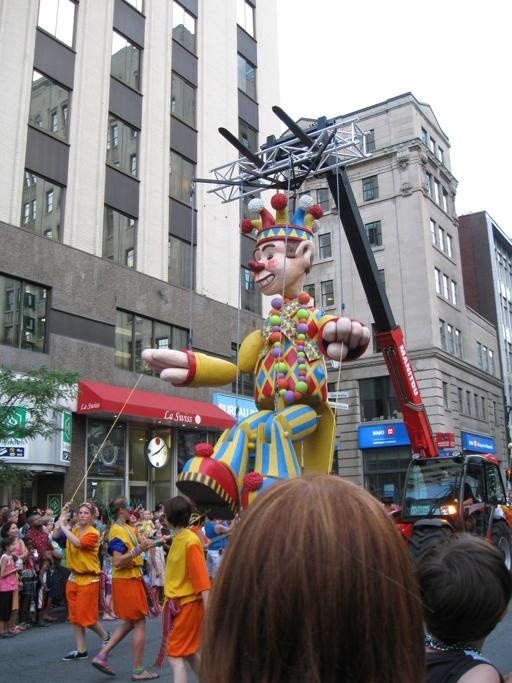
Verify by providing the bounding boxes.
[22,569,38,581]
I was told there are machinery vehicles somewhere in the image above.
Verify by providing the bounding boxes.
[216,100,512,581]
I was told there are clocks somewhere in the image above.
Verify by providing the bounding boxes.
[144,436,170,470]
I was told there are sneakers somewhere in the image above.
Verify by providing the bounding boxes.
[0,614,58,638]
[102,615,116,620]
[62,649,89,661]
[102,631,113,656]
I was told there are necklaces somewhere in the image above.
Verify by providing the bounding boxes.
[424,632,485,656]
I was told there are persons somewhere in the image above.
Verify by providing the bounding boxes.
[138,192,372,518]
[193,469,428,682]
[438,467,475,508]
[51,498,110,660]
[162,495,211,682]
[414,530,512,682]
[0,497,240,640]
[91,496,168,680]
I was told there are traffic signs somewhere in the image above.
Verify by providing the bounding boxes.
[327,390,350,397]
[327,400,350,411]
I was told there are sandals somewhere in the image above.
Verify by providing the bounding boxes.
[91,656,116,676]
[132,670,160,681]
